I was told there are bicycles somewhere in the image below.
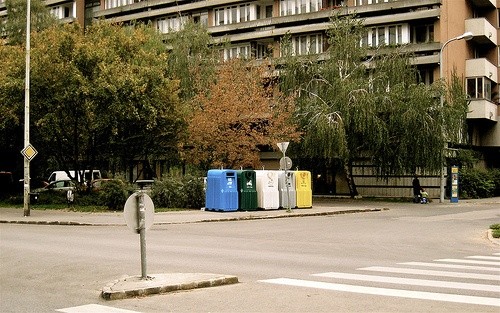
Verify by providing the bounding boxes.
[420,198,429,204]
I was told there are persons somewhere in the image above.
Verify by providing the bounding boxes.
[413,174,423,204]
[421,189,432,203]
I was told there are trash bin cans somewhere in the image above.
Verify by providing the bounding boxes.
[206,170,312,209]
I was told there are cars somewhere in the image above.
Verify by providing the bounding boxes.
[0,170,207,196]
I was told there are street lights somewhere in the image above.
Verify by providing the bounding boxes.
[440,32,473,202]
